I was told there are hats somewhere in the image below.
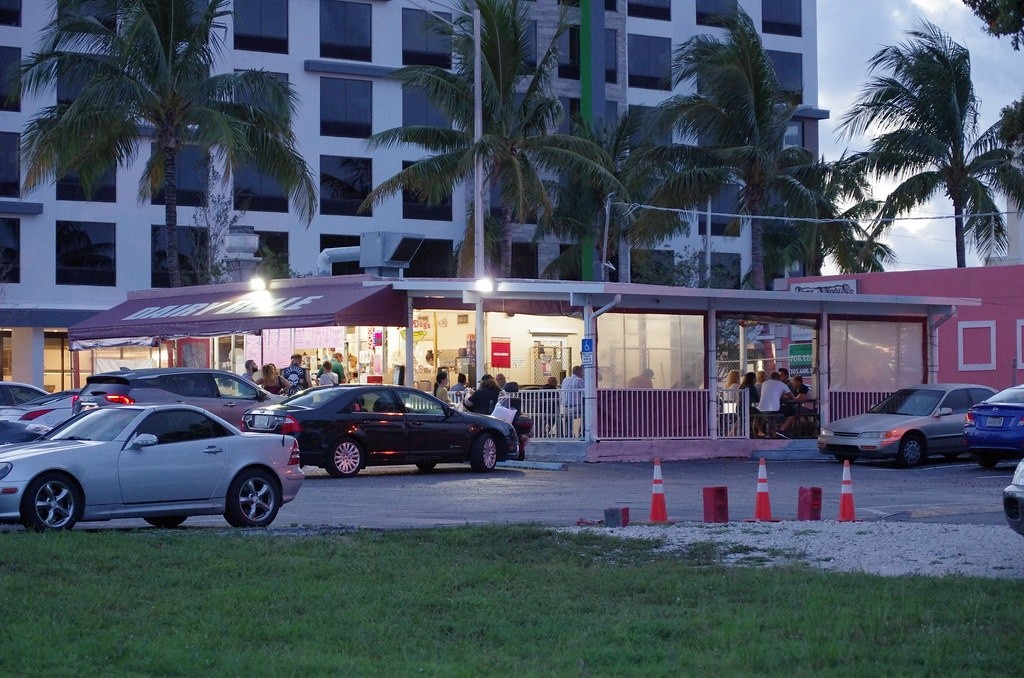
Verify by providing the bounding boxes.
[481,374,494,380]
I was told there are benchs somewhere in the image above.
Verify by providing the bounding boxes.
[738,414,783,438]
[796,412,821,436]
[523,412,584,437]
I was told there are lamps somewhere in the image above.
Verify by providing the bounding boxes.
[476,276,496,294]
[250,276,268,290]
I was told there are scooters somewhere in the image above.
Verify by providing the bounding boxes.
[511,416,533,462]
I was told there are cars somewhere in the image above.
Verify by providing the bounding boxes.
[0,402,305,534]
[1002,457,1024,537]
[817,383,1000,470]
[0,380,83,446]
[240,382,520,479]
[963,383,1024,467]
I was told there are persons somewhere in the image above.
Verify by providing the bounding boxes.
[434,371,452,404]
[317,352,357,386]
[461,373,533,450]
[724,367,809,439]
[559,365,585,438]
[426,350,435,372]
[238,359,291,399]
[535,377,560,434]
[670,372,697,388]
[628,369,654,389]
[282,353,312,396]
[449,373,466,403]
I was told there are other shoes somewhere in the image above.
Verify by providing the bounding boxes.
[774,430,789,439]
[758,432,765,437]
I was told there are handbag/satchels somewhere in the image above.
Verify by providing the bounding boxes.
[489,393,517,424]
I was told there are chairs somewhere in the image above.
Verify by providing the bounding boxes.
[359,396,368,412]
[373,397,388,412]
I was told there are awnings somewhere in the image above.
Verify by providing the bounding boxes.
[66,283,409,352]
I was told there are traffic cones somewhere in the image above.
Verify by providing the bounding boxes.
[836,459,865,521]
[743,457,782,522]
[649,457,669,523]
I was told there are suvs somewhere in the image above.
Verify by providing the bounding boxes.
[71,367,285,431]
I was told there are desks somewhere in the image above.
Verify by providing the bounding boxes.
[780,398,817,437]
